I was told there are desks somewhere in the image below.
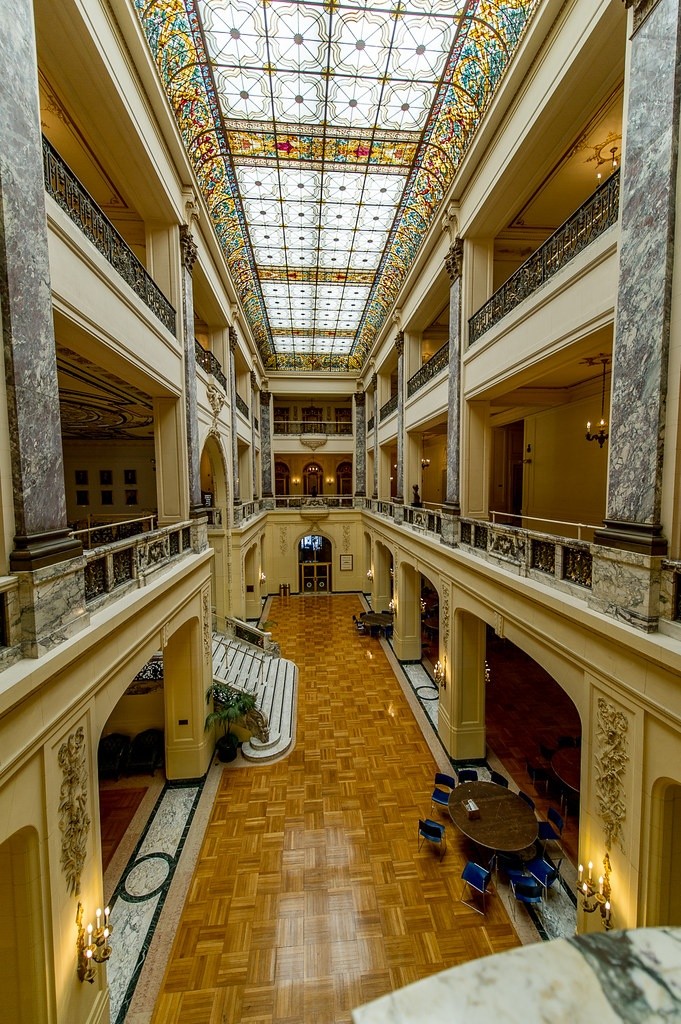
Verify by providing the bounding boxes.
[360,613,393,627]
[550,746,581,794]
[424,615,439,641]
[448,781,540,851]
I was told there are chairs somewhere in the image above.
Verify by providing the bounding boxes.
[417,819,447,863]
[98,728,164,782]
[430,770,564,923]
[353,610,393,640]
[526,736,582,829]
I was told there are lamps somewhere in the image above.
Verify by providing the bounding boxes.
[433,656,446,691]
[585,359,609,448]
[595,147,617,188]
[485,661,491,684]
[421,435,431,470]
[260,573,266,584]
[367,570,373,584]
[575,854,614,932]
[389,599,397,618]
[306,454,318,473]
[76,901,113,985]
[402,598,426,613]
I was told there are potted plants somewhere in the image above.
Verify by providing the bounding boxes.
[204,681,257,763]
[261,619,278,632]
